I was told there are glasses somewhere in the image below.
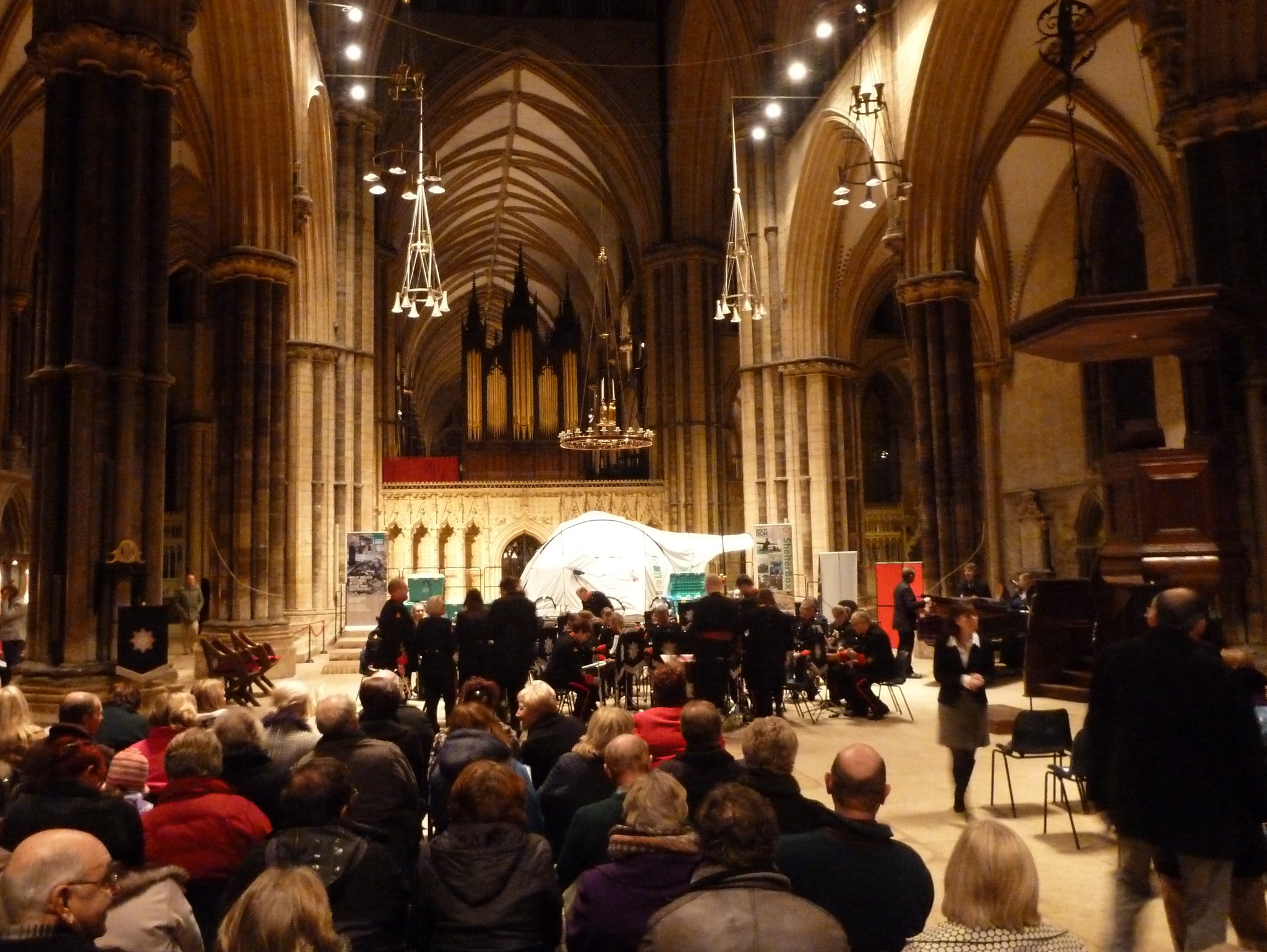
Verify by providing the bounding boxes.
[68,869,119,890]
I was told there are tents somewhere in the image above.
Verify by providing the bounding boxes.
[519,510,754,617]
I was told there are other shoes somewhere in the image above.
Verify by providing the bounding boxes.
[806,686,817,700]
[905,671,923,679]
[868,705,889,720]
[821,699,840,706]
[844,709,866,717]
[954,797,965,813]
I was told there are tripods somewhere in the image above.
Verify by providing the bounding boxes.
[803,677,844,722]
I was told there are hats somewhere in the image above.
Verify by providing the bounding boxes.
[106,747,149,792]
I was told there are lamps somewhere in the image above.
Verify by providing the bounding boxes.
[557,154,655,449]
[365,0,446,199]
[324,75,451,318]
[713,94,820,322]
[832,4,912,208]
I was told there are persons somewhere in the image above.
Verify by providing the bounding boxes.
[0,669,935,952]
[892,567,931,679]
[1070,588,1267,952]
[787,598,900,719]
[358,576,540,722]
[1009,572,1030,605]
[932,600,995,812]
[959,565,991,600]
[902,818,1088,952]
[0,585,28,670]
[545,587,684,718]
[687,573,797,723]
[174,574,204,654]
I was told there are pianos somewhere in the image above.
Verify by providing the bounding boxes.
[914,594,1031,667]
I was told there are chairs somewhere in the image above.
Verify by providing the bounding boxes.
[991,708,1089,849]
[779,650,914,723]
[202,628,280,710]
[530,661,575,716]
